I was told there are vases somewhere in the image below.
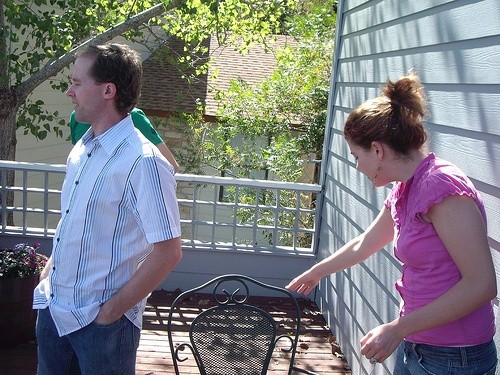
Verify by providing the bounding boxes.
[0,275,39,348]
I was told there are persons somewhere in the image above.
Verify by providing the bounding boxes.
[32,44,180,375]
[69,105,179,173]
[285,72,497,375]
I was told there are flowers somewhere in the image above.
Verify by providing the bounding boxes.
[0,241,49,278]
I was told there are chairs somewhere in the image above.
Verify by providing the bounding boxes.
[167,273,316,375]
